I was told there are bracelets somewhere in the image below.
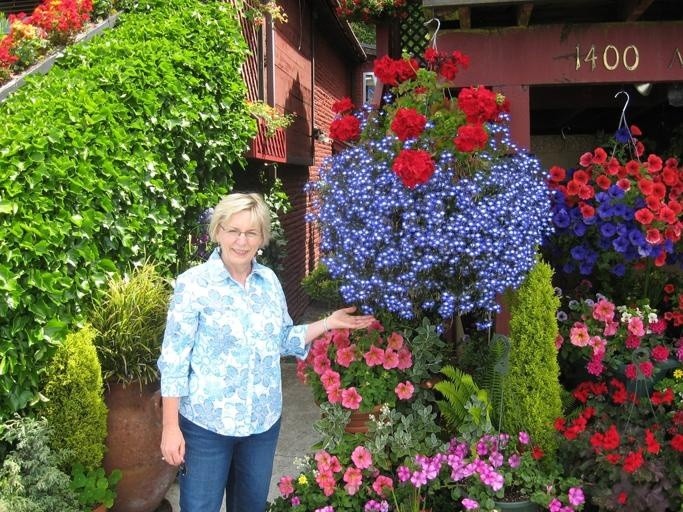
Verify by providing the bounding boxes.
[323,317,328,332]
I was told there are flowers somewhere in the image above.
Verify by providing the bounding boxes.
[246,44,683,512]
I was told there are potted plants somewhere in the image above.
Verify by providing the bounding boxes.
[67,462,123,512]
[86,257,184,511]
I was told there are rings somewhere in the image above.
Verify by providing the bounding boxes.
[161,456,165,461]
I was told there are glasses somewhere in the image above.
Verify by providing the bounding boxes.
[219,224,262,240]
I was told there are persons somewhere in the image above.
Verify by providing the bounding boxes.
[157,190,377,512]
